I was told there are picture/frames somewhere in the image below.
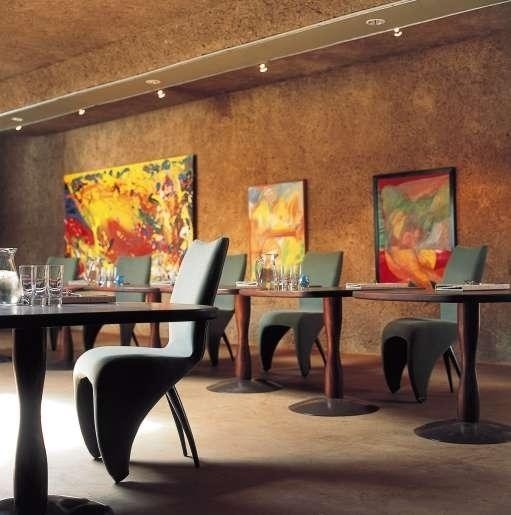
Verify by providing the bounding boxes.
[373,167,456,289]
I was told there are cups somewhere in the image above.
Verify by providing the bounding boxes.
[289,264,302,291]
[100,267,111,287]
[29,265,50,305]
[19,265,35,307]
[114,266,121,285]
[281,264,293,289]
[47,265,63,305]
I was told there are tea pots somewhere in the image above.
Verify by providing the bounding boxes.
[0,248,19,307]
[254,253,279,291]
[85,257,106,288]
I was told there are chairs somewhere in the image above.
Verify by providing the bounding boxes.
[72,236,230,485]
[258,251,344,379]
[42,256,80,351]
[84,256,151,351]
[205,253,246,368]
[380,245,489,404]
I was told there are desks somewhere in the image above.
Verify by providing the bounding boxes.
[0,303,218,515]
[238,286,425,417]
[42,293,116,363]
[160,286,322,393]
[83,284,159,349]
[352,288,511,443]
